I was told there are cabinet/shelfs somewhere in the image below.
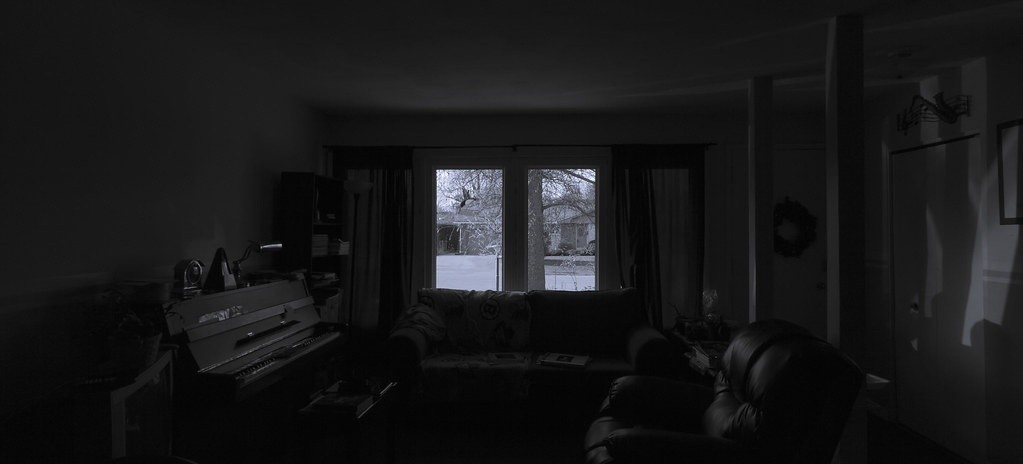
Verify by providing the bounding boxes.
[274,171,349,322]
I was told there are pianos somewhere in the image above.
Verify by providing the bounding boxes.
[115,271,352,464]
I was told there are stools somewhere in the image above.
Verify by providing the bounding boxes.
[295,374,397,464]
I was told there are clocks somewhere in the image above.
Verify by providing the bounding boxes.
[173,258,206,299]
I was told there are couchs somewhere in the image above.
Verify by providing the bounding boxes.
[584,317,863,464]
[379,287,673,410]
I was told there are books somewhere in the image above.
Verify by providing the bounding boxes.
[311,233,351,257]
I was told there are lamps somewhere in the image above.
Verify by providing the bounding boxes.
[232,240,283,287]
